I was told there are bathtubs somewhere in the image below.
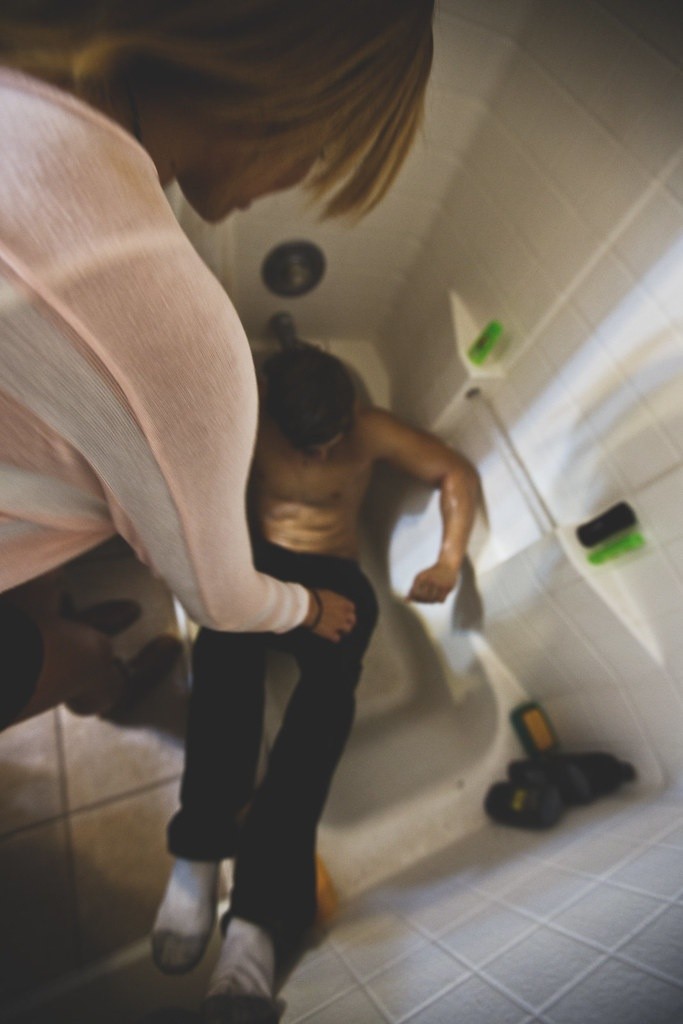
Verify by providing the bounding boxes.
[135,335,559,907]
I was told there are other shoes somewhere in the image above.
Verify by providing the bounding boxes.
[66,598,141,638]
[100,635,183,728]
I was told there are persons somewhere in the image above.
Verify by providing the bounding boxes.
[147,342,480,1000]
[0,0,433,732]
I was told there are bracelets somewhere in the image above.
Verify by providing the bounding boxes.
[310,591,323,628]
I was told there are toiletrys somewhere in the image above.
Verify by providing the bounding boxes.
[463,318,506,366]
[577,501,645,566]
